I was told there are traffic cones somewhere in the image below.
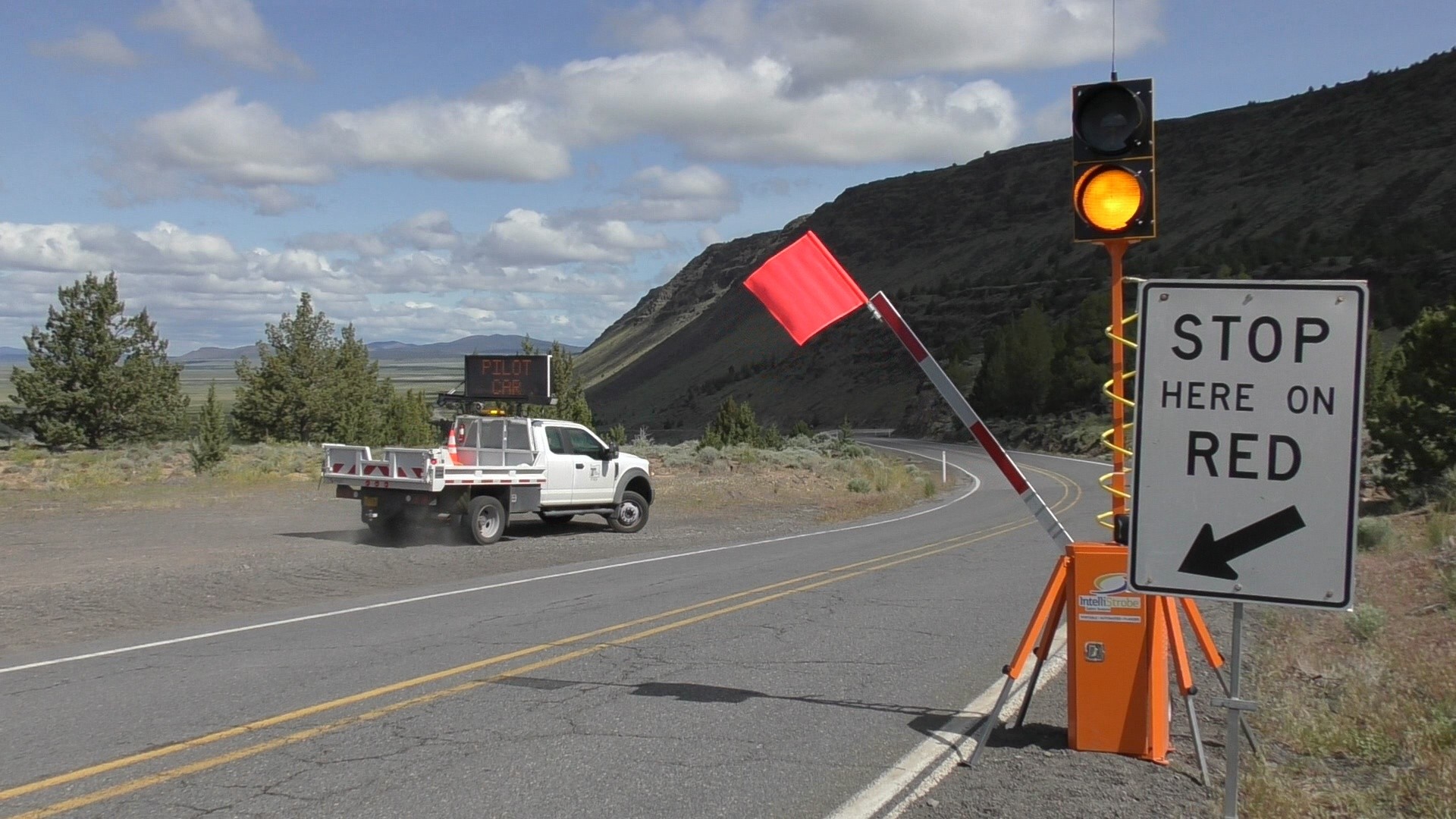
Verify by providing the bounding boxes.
[446,429,464,466]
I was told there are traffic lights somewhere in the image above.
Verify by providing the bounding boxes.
[1069,77,1156,242]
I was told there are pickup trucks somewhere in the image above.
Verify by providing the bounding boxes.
[318,407,655,547]
